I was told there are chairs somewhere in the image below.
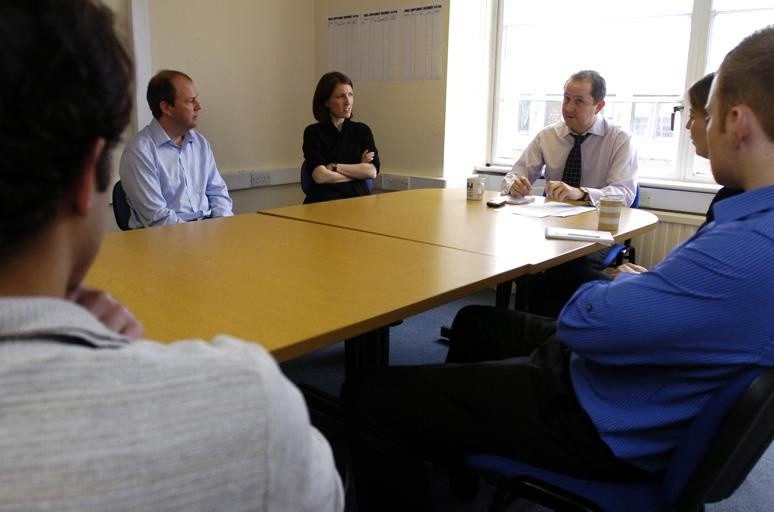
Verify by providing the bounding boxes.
[301,160,373,204]
[112,181,132,232]
[484,371,774,512]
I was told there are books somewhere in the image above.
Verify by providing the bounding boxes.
[545,227,614,247]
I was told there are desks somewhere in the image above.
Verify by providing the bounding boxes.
[84,213,529,370]
[257,188,658,340]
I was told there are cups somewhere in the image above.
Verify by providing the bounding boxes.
[596,197,624,233]
[467,177,485,201]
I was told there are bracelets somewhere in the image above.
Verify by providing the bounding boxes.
[331,162,337,172]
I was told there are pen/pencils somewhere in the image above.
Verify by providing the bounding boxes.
[532,187,545,190]
[568,234,600,238]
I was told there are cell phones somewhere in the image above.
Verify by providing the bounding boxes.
[487,197,506,206]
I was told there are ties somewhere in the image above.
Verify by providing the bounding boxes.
[561,132,591,188]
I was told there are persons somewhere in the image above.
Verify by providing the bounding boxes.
[522,71,745,320]
[302,70,381,203]
[337,27,772,511]
[0,0,350,511]
[119,67,237,227]
[500,69,640,317]
